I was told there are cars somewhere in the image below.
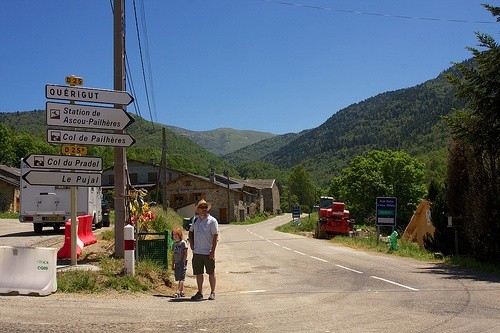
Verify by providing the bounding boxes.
[101,200,111,226]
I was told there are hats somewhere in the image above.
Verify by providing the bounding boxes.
[194,198,212,212]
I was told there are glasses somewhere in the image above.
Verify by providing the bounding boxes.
[199,205,208,209]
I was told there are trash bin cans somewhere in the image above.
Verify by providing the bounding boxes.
[136,230,168,269]
[183,218,191,228]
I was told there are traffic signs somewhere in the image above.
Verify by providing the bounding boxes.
[45,102,135,134]
[22,154,104,172]
[45,84,135,109]
[46,129,137,149]
[20,170,103,190]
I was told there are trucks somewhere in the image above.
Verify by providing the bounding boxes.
[20,157,105,235]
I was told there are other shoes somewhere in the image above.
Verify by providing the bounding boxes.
[172,290,185,298]
[208,293,215,300]
[191,292,203,300]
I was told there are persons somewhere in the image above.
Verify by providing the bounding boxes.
[171,228,188,297]
[189,199,219,300]
